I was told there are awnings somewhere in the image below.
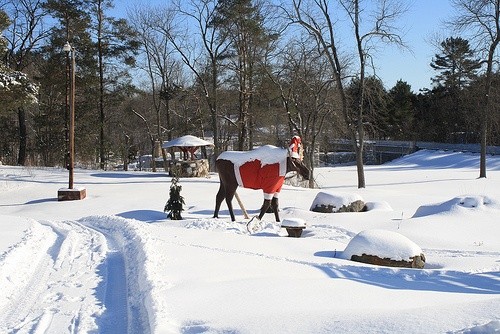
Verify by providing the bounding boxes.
[162,134,215,176]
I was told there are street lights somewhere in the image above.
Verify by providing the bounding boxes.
[62,41,72,165]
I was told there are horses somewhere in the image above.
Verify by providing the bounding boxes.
[212,146,310,222]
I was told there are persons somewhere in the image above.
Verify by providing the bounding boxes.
[289,135,302,160]
